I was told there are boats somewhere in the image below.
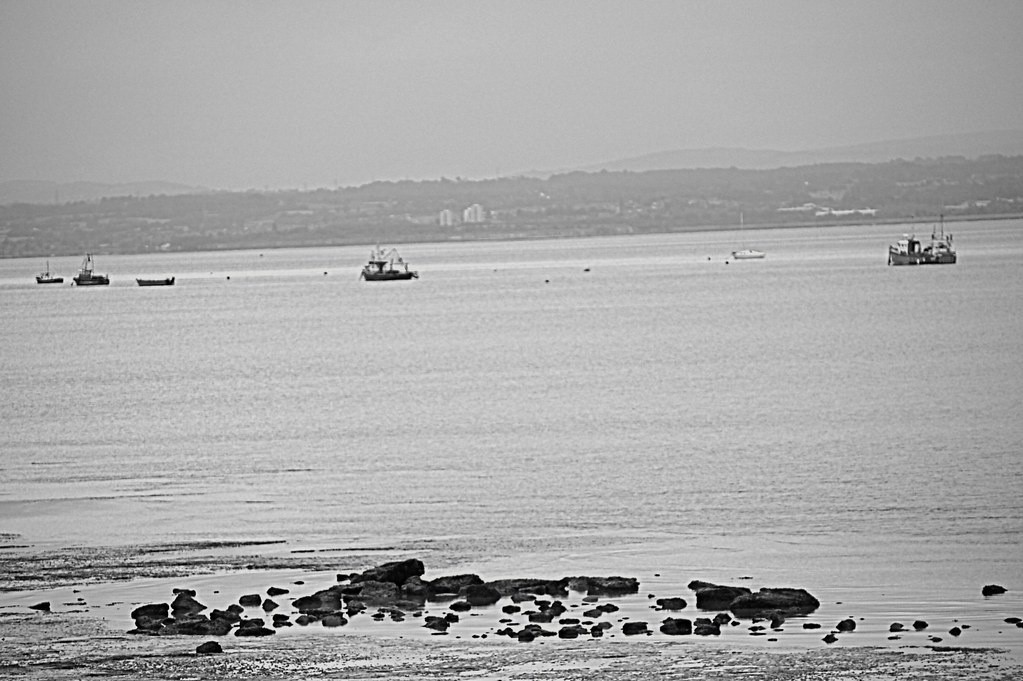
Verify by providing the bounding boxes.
[134,277,176,288]
[72,250,111,286]
[357,241,420,280]
[884,212,958,264]
[34,257,64,283]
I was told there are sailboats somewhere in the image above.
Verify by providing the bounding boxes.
[729,213,765,260]
